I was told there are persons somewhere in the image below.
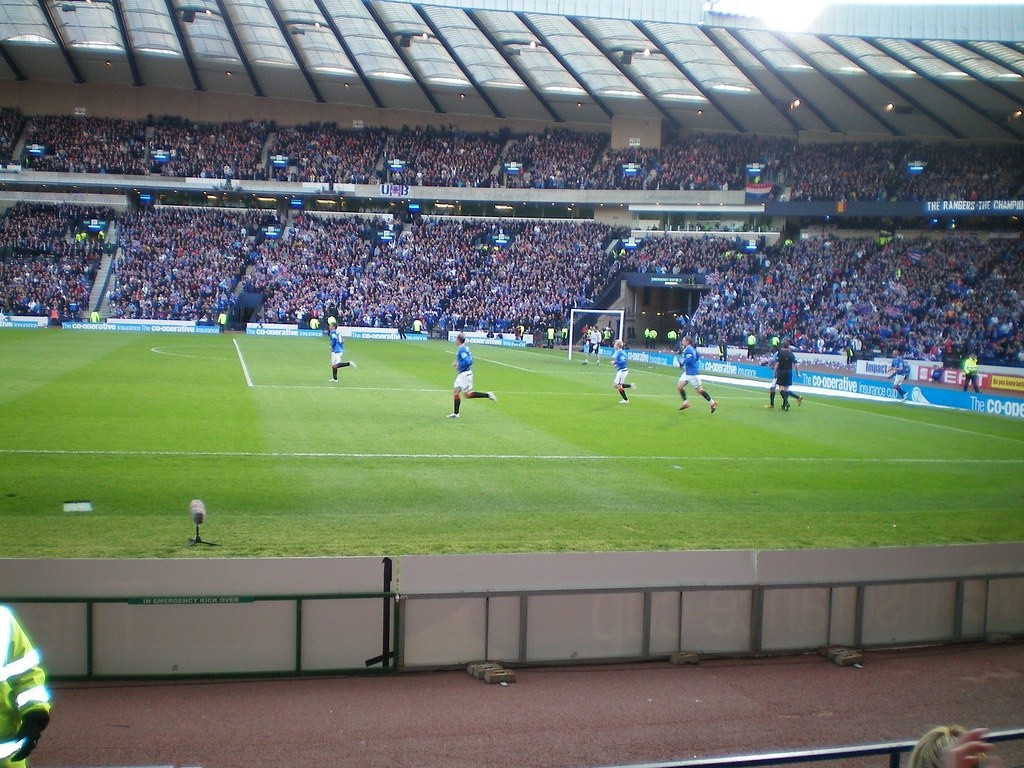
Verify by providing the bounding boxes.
[961,354,980,392]
[773,341,804,411]
[446,334,497,418]
[0,106,1024,367]
[905,725,1006,767]
[610,340,637,404]
[329,323,357,382]
[0,604,51,768]
[677,336,718,414]
[886,351,909,404]
[765,346,791,410]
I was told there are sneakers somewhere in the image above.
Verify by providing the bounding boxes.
[679,403,690,410]
[798,396,802,406]
[487,392,497,402]
[596,361,599,366]
[329,379,338,382]
[619,399,629,403]
[447,413,459,418]
[583,360,589,365]
[765,404,774,408]
[630,383,637,392]
[710,402,717,413]
[348,361,356,368]
[901,391,908,402]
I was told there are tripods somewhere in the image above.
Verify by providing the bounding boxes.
[189,523,223,547]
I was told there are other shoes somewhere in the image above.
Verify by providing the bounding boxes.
[780,403,789,411]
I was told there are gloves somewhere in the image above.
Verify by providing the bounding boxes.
[11,710,49,761]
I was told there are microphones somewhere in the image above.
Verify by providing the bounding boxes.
[188,499,205,524]
